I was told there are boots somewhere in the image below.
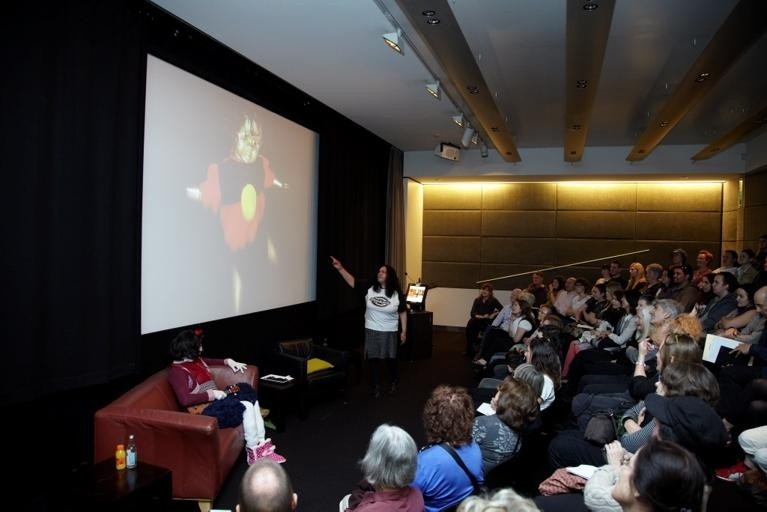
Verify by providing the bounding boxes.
[260,438,286,464]
[246,441,263,466]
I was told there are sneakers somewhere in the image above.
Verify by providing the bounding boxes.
[715,463,748,481]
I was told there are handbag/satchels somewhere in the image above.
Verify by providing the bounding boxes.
[585,409,618,445]
[591,336,618,349]
[539,467,587,496]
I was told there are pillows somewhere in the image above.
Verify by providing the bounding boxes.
[306,357,335,375]
[279,338,312,359]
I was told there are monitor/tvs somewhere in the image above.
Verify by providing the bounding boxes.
[403,282,428,306]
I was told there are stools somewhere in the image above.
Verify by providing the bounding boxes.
[258,374,299,418]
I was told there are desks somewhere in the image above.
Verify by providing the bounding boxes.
[95,456,173,512]
[399,310,433,359]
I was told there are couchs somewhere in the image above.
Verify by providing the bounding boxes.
[93,358,260,512]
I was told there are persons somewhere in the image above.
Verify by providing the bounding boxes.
[168,329,287,464]
[459,247,765,511]
[330,255,408,400]
[233,457,298,511]
[352,423,425,512]
[338,384,485,512]
[187,112,290,316]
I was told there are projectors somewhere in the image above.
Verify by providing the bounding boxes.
[434,142,461,163]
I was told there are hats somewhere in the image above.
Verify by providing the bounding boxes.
[644,393,729,466]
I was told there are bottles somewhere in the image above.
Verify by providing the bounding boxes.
[116,445,126,470]
[126,435,137,468]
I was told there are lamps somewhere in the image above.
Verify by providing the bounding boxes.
[479,142,488,158]
[472,132,478,144]
[424,80,441,100]
[383,29,401,52]
[453,112,463,128]
[460,127,474,149]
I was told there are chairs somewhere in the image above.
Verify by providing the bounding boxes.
[268,331,352,420]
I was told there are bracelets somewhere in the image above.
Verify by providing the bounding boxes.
[337,266,345,269]
